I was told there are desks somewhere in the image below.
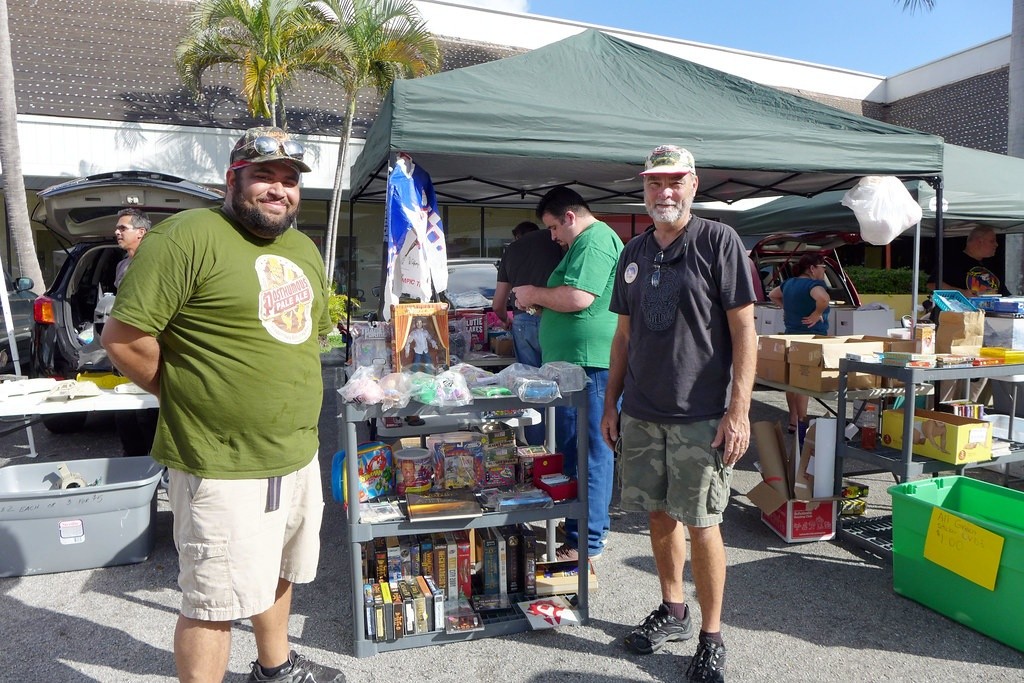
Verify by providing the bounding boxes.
[756,376,935,485]
[0,389,161,416]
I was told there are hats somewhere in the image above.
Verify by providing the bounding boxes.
[638,145,696,176]
[229,125,312,173]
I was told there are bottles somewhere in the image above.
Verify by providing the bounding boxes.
[861,406,877,449]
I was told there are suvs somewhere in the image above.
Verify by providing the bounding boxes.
[747,231,862,309]
[13,170,225,436]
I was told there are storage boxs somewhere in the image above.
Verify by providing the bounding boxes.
[887,475,1024,651]
[853,397,897,440]
[746,461,870,543]
[755,290,1024,392]
[882,408,992,466]
[390,302,450,376]
[0,456,165,579]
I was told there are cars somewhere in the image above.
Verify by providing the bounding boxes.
[372,258,499,320]
[0,266,40,374]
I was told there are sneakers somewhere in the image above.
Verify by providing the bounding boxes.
[624,603,695,655]
[539,543,605,562]
[558,521,608,546]
[247,650,347,683]
[685,637,727,683]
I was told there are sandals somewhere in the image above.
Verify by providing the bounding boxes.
[787,424,797,434]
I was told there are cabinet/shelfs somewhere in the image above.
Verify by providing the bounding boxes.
[343,389,589,657]
[834,357,1024,564]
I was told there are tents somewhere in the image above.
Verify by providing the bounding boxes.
[743,143,1024,294]
[347,28,945,327]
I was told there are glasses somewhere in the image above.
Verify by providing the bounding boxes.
[650,248,664,289]
[809,264,827,268]
[113,225,140,231]
[237,136,305,161]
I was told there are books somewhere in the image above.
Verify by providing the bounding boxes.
[882,350,1005,419]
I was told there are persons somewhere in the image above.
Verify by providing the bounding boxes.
[602,144,759,683]
[769,254,830,433]
[99,126,347,683]
[511,186,627,561]
[926,223,1011,295]
[492,221,565,446]
[404,317,439,373]
[114,208,159,457]
